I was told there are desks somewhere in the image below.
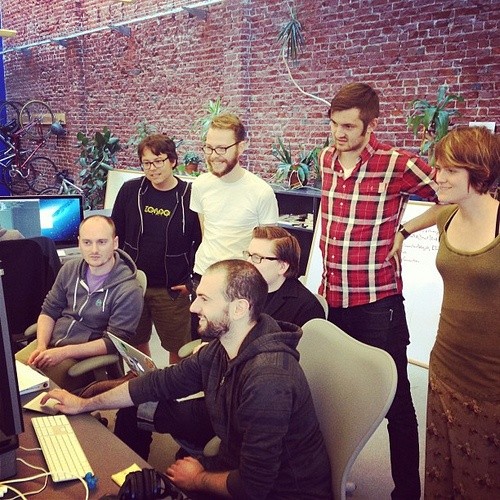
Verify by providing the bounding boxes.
[0,367,186,500]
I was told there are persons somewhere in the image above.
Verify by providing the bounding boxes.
[111,134,202,365]
[41,257,334,500]
[193,226,326,355]
[15,214,144,389]
[189,113,279,344]
[421,125,500,500]
[304,82,455,500]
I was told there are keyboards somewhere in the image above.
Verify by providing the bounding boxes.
[31,414,95,483]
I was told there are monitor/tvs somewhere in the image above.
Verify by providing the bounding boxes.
[0,278,25,437]
[0,194,84,246]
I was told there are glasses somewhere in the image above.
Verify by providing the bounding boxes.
[142,157,168,171]
[243,251,278,264]
[202,141,238,155]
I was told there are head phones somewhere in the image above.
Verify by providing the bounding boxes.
[118,467,190,500]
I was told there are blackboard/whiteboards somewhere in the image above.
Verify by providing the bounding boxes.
[397,199,444,369]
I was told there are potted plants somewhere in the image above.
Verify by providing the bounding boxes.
[266,136,318,188]
[402,87,465,200]
[182,152,205,174]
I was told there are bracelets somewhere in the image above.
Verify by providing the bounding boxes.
[398,224,411,239]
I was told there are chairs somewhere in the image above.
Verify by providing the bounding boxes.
[24,269,147,395]
[297,319,397,500]
